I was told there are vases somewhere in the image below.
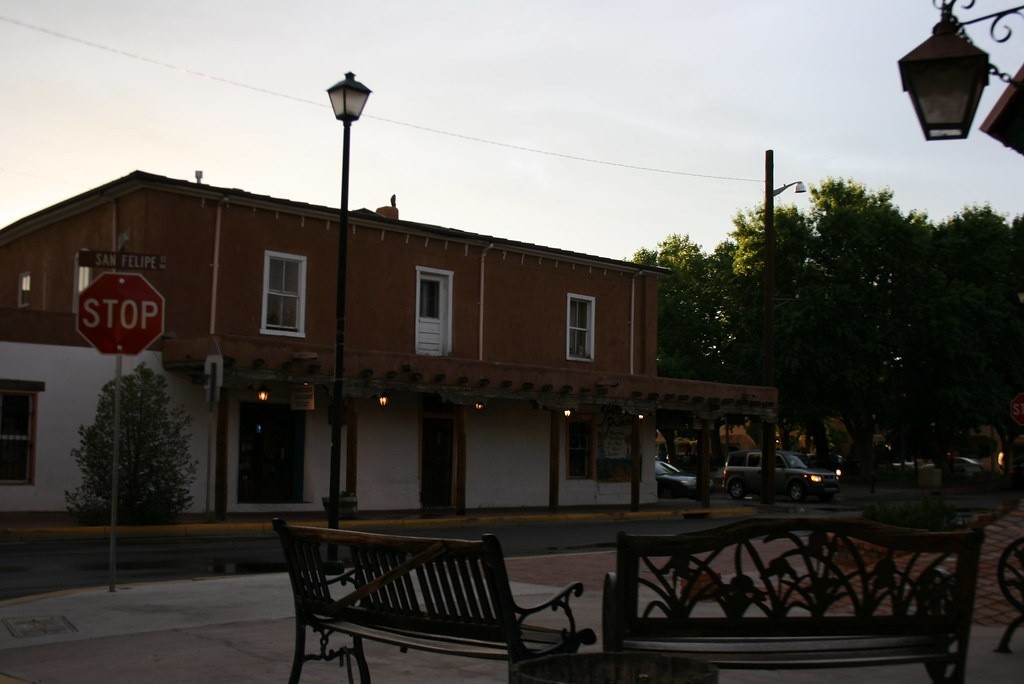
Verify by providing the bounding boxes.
[511,648,719,684]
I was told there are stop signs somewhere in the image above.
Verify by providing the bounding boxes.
[73,270,167,357]
[1011,393,1024,426]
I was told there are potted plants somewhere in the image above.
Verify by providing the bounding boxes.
[321,490,358,519]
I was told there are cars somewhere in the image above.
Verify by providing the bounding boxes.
[656,461,715,502]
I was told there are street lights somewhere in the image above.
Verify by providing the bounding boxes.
[314,71,373,576]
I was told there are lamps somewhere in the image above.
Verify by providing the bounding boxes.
[257,384,270,401]
[378,393,389,407]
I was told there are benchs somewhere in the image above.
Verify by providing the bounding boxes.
[603,516,985,684]
[993,536,1024,654]
[272,518,597,684]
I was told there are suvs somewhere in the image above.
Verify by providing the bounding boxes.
[722,452,839,504]
